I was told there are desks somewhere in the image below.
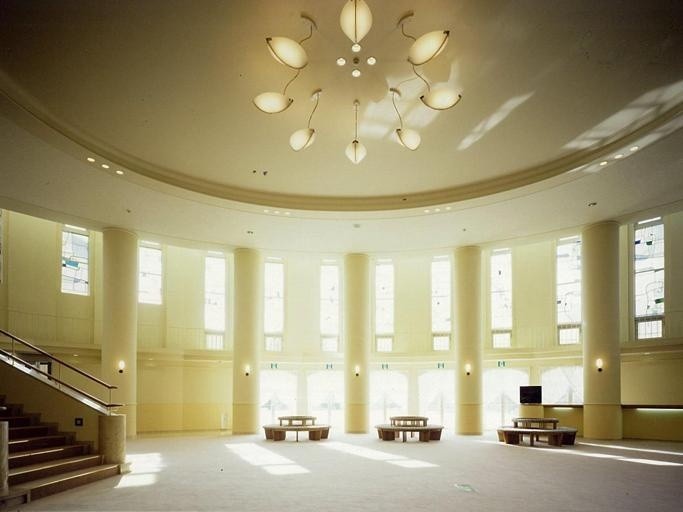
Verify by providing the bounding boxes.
[388,416,430,443]
[511,417,560,446]
[277,416,318,442]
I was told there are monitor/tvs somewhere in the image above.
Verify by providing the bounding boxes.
[519,385,542,404]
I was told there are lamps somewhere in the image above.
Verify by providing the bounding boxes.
[253,0,464,165]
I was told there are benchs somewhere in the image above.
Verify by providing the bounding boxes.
[497,425,578,447]
[263,422,331,441]
[375,423,444,443]
